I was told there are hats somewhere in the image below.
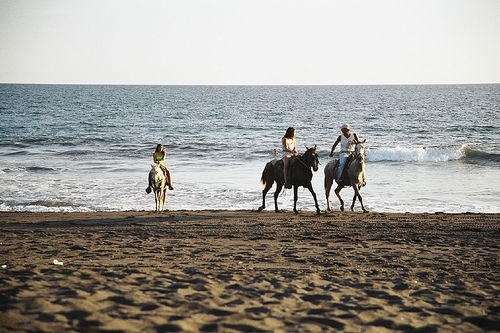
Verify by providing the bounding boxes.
[339,124,350,130]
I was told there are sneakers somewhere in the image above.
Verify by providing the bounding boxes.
[147,188,150,193]
[169,186,174,190]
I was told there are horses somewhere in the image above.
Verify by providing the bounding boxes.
[323,139,370,212]
[257,144,322,214]
[150,163,167,213]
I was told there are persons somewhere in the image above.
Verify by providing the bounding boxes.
[329,122,359,184]
[148,143,174,190]
[281,126,298,187]
